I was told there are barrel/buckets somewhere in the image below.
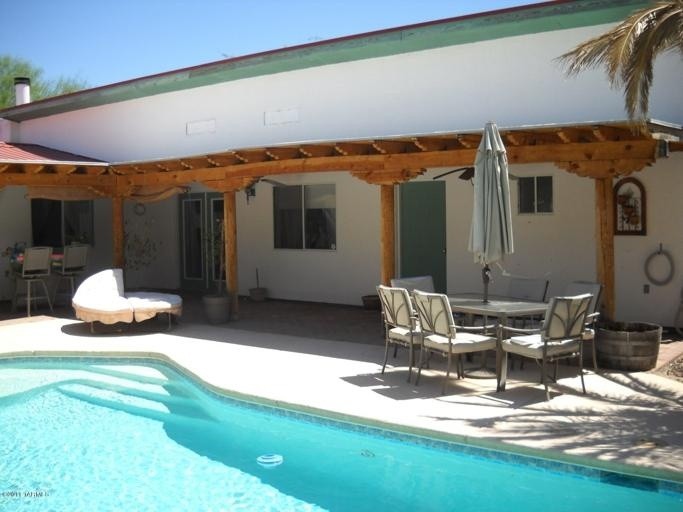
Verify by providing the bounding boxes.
[592,321,663,373]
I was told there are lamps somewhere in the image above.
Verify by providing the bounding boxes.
[244,180,257,207]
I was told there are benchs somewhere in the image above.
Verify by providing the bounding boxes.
[67,264,186,338]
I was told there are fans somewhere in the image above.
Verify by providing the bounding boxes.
[432,166,519,184]
[247,175,287,192]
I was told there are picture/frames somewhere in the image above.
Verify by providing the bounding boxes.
[611,177,649,236]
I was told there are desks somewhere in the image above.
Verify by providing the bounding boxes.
[432,290,559,381]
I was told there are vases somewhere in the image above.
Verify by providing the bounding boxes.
[199,290,236,330]
[248,287,270,305]
[361,291,386,312]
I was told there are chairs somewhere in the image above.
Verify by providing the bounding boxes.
[501,274,555,375]
[499,293,597,403]
[412,286,503,398]
[376,284,441,388]
[47,240,94,308]
[562,278,606,378]
[9,246,59,324]
[385,273,438,299]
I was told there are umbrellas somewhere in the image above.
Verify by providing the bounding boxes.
[468,120,514,372]
